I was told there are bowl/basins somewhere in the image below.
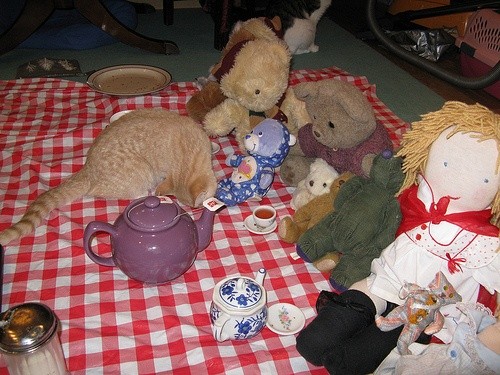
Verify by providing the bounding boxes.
[253,205,277,228]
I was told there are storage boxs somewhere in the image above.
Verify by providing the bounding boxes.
[454,7,500,67]
[385,0,477,44]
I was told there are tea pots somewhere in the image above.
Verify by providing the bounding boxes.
[84,195,225,285]
[210,268,269,342]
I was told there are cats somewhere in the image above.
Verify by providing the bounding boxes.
[0,108,218,247]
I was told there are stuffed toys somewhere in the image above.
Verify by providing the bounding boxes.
[188,14,500,375]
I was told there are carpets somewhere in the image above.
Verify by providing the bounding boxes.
[0,66,412,375]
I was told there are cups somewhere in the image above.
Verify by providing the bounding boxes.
[0,302,70,375]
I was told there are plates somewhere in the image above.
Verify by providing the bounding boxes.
[243,214,278,234]
[86,64,172,96]
[210,141,221,155]
[266,302,305,336]
[110,110,135,124]
